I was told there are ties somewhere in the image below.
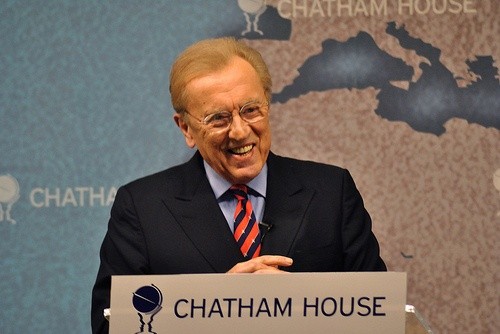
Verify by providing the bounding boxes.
[228,183,262,267]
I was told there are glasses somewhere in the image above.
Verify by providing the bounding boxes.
[181,95,270,133]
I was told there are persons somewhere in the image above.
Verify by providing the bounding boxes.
[90,35,395,334]
